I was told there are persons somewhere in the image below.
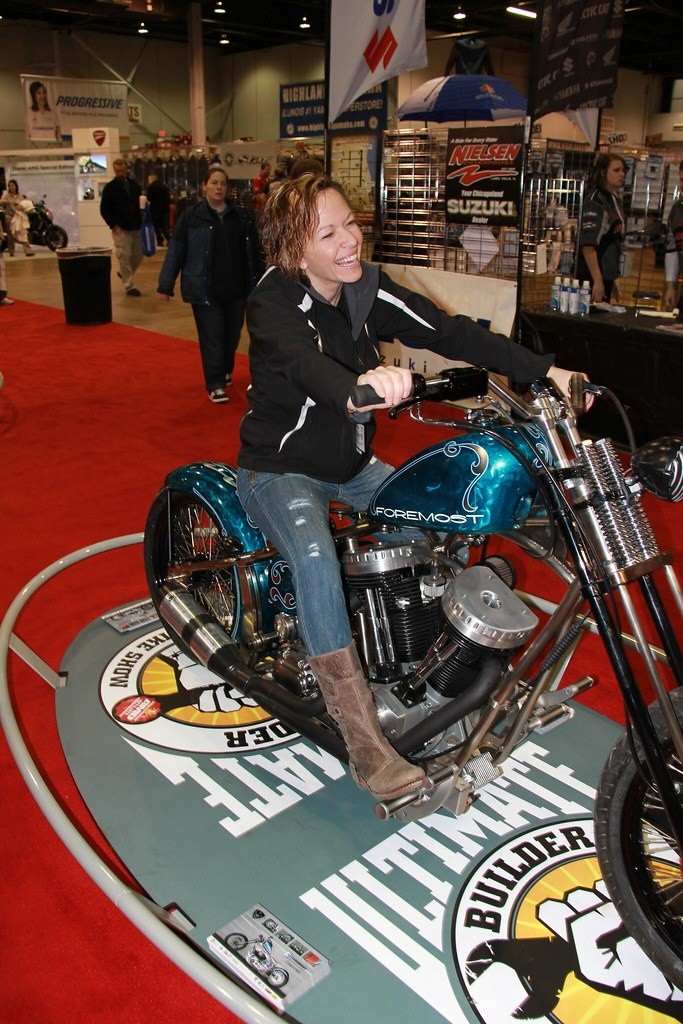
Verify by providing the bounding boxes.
[575,153,630,303]
[0,220,13,304]
[156,164,267,402]
[253,156,289,209]
[0,180,35,257]
[147,175,190,246]
[664,160,683,324]
[236,172,595,801]
[100,159,143,297]
[29,81,61,140]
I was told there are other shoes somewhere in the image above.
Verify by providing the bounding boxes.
[26,252,35,256]
[10,253,14,256]
[117,272,122,281]
[126,287,140,296]
[2,297,14,304]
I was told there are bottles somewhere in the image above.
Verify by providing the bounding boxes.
[568,279,580,314]
[578,281,592,316]
[550,276,562,311]
[559,278,570,312]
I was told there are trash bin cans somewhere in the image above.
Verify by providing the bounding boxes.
[56,245,113,324]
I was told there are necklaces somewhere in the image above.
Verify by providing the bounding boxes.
[304,270,342,306]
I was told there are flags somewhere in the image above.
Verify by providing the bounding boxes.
[327,0,429,129]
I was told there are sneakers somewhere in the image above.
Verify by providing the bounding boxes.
[208,387,229,402]
[226,374,232,385]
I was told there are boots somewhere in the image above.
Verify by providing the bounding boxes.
[305,639,425,801]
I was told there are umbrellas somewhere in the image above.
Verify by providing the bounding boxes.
[396,74,527,128]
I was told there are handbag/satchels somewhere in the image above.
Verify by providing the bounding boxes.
[236,205,260,296]
[141,208,156,256]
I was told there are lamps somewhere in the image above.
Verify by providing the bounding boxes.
[136,22,149,34]
[298,17,311,29]
[506,5,538,19]
[219,33,229,45]
[451,5,466,20]
[214,1,226,14]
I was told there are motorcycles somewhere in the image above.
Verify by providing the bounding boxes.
[0,194,68,251]
[142,366,683,993]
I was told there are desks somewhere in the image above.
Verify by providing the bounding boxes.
[518,298,683,455]
